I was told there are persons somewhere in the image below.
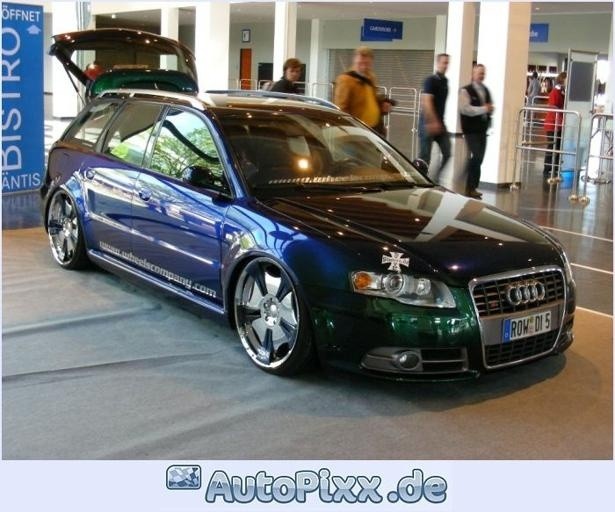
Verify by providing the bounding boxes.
[545,78,553,94]
[525,72,541,122]
[264,59,303,94]
[536,175,557,234]
[543,72,567,175]
[458,64,494,197]
[335,45,392,140]
[418,53,451,182]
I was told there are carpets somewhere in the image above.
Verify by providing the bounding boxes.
[1,221,612,459]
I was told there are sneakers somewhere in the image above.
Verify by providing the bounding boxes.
[466,187,483,199]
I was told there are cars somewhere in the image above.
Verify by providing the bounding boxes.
[37,26,578,377]
